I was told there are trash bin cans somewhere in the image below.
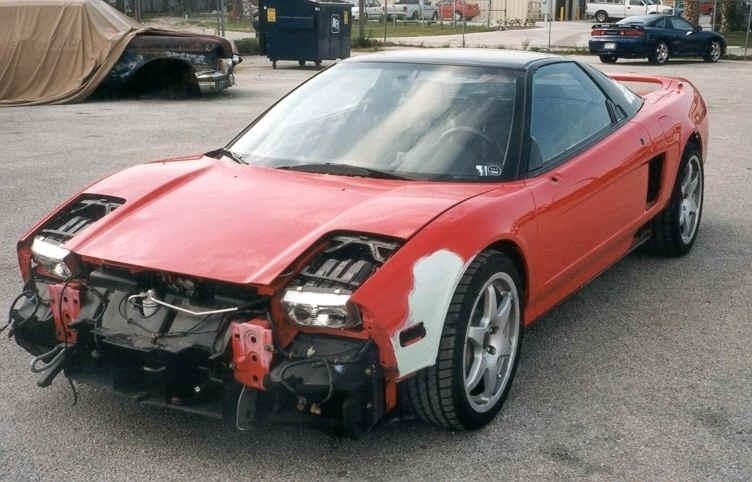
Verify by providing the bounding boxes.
[258,1,354,69]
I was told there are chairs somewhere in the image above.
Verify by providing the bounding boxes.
[459,92,512,172]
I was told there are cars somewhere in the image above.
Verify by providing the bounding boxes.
[588,14,728,66]
[436,0,481,21]
[14,45,711,432]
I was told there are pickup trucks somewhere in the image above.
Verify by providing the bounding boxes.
[587,0,673,24]
[352,0,439,24]
